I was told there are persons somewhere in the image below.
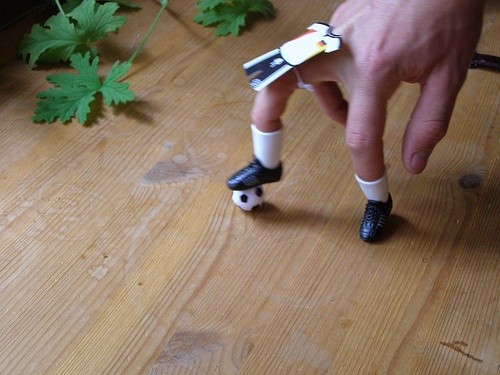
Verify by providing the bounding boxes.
[225,0,490,244]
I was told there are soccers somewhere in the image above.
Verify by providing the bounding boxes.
[231,185,264,211]
